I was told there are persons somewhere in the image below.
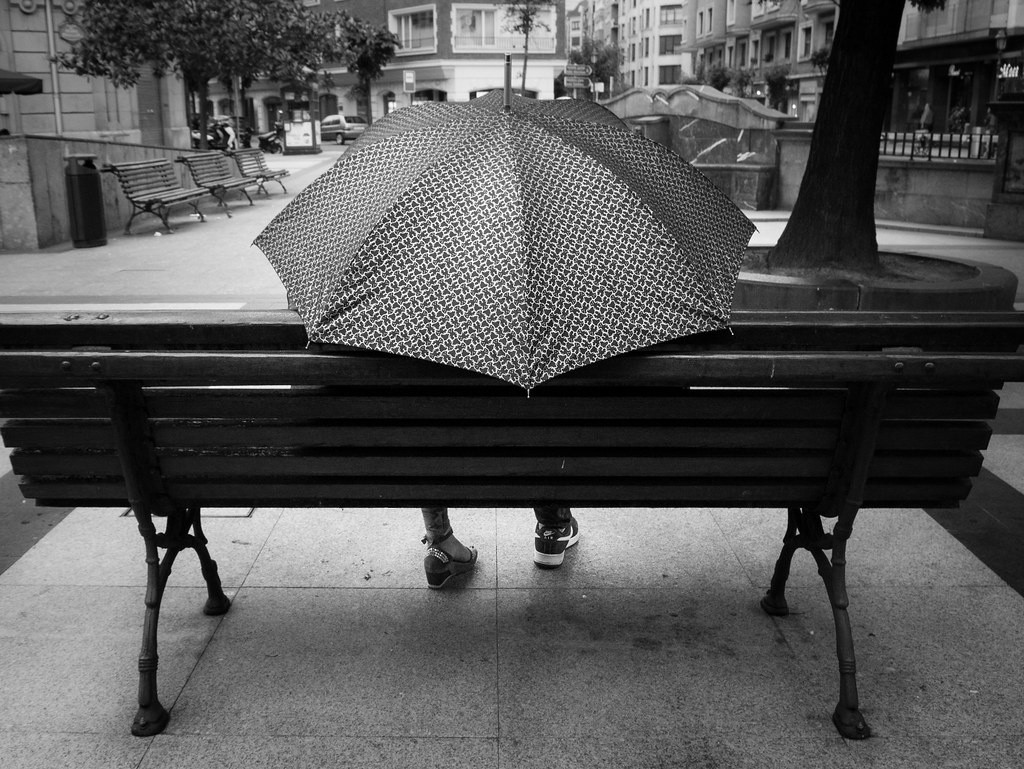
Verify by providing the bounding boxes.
[417,505,478,589]
[916,103,934,150]
[188,114,237,151]
[532,505,581,571]
[982,105,999,158]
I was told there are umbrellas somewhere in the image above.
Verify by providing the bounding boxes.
[251,52,762,399]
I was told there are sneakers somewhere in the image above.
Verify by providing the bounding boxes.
[532,517,580,565]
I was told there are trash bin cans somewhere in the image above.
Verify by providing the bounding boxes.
[62,154,107,248]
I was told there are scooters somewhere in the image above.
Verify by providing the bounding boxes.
[190,111,240,156]
[238,123,284,154]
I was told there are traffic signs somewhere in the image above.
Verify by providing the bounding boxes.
[564,64,593,89]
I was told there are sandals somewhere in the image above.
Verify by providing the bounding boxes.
[424,545,478,589]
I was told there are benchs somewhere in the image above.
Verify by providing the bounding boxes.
[223,149,290,196]
[173,151,257,219]
[102,158,211,237]
[0,310,1024,746]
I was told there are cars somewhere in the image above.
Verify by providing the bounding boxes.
[320,114,368,145]
